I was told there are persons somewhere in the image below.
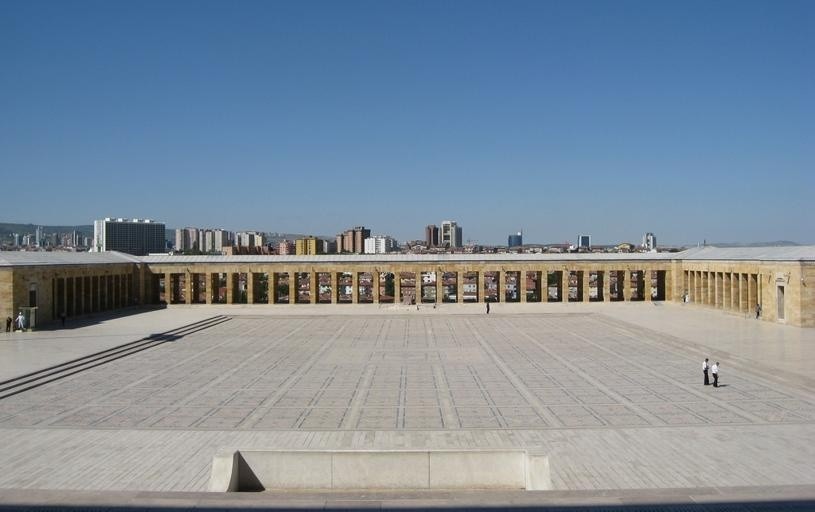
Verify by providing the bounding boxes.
[15,312,26,330]
[702,358,711,385]
[6,317,12,332]
[755,303,761,319]
[711,362,719,387]
